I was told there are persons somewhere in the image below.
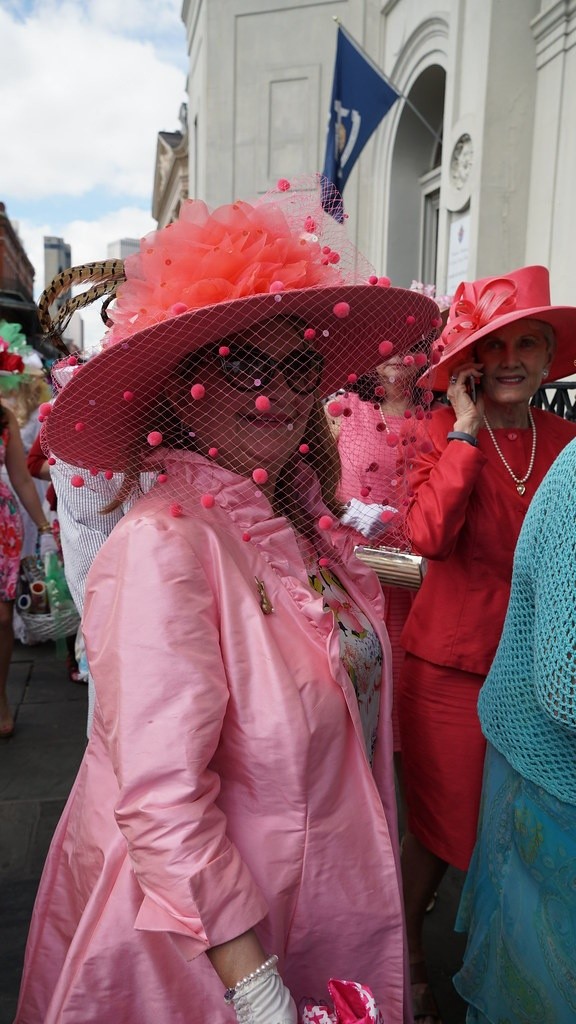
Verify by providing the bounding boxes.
[0,197,576,1024]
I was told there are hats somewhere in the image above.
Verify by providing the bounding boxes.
[37,174,444,582]
[417,266,576,393]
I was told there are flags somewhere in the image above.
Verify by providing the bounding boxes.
[318,30,399,221]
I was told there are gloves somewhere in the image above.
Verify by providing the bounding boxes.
[233,967,297,1024]
[338,494,397,540]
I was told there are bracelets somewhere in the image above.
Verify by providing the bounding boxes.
[38,523,52,532]
[224,954,279,1000]
[446,430,477,446]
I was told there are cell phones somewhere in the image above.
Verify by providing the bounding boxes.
[464,376,477,404]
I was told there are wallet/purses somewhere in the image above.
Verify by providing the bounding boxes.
[354,546,427,589]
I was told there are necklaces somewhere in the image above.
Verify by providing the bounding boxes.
[482,407,536,495]
[377,403,390,435]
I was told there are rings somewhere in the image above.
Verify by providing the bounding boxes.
[451,377,456,384]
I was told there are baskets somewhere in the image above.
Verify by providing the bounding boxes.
[20,553,83,640]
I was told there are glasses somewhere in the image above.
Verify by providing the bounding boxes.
[185,344,325,395]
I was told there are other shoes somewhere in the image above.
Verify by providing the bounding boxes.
[0,717,14,734]
[66,657,89,683]
